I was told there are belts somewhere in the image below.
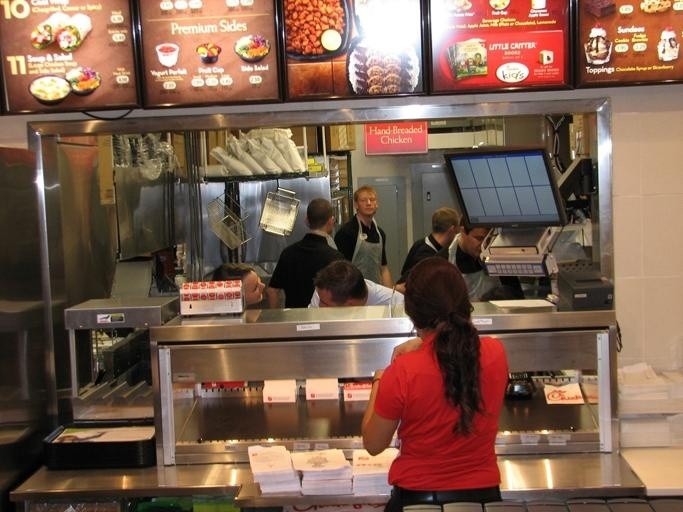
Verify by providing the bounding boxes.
[395,486,501,501]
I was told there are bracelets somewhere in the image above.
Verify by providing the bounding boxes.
[371,377,379,384]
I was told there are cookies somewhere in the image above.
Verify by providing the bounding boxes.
[639,1,672,13]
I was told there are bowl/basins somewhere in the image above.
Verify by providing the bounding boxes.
[232,39,271,63]
[70,69,101,97]
[193,44,222,64]
[28,74,72,106]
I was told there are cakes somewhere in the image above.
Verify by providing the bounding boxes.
[585,24,610,65]
[657,26,680,62]
[585,0,616,17]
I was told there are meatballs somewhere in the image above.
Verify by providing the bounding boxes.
[366,46,401,94]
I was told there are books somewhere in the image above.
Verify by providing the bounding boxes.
[245,445,399,497]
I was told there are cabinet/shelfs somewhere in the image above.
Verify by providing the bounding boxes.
[328,153,354,227]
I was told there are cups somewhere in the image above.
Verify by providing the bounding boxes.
[154,43,180,68]
[657,43,682,62]
[583,41,614,65]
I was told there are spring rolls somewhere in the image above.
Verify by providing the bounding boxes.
[55,14,92,53]
[30,12,71,48]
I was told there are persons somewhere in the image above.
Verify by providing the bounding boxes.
[435,212,525,302]
[360,258,508,511]
[395,208,465,293]
[307,260,404,310]
[211,263,264,307]
[335,186,394,290]
[266,198,345,310]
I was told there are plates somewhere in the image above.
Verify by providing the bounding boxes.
[283,0,351,63]
[343,34,422,93]
[495,62,530,83]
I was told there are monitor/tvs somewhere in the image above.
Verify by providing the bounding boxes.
[444,145,569,230]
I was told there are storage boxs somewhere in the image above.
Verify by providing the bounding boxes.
[330,125,356,152]
